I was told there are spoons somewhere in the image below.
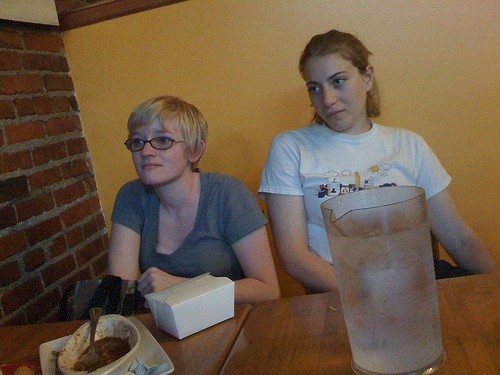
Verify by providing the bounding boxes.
[79,307,103,371]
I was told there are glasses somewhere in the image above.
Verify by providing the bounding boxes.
[124,136,185,152]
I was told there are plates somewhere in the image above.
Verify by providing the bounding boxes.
[40,314,175,375]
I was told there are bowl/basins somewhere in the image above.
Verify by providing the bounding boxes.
[57,314,141,375]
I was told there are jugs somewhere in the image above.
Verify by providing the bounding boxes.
[321,184,448,375]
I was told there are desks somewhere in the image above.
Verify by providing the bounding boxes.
[0,272,500,375]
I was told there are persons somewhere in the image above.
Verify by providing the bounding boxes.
[258,30,500,295]
[108,95,280,310]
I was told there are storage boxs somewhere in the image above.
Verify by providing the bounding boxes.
[143,271,235,340]
